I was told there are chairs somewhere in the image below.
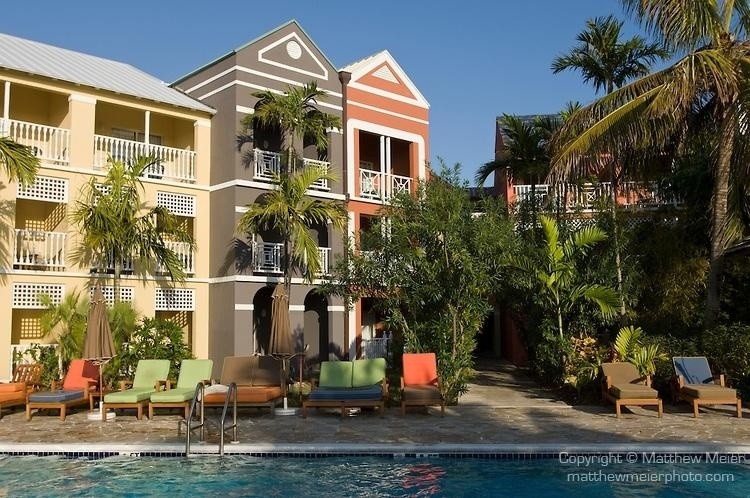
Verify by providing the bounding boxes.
[25,359,101,422]
[600,361,663,418]
[101,359,171,422]
[400,352,445,418]
[0,363,44,419]
[671,356,743,418]
[147,358,213,420]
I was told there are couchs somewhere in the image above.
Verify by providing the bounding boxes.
[197,354,291,418]
[302,357,390,419]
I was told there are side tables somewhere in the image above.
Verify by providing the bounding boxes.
[89,389,124,414]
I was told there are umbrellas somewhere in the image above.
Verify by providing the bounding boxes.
[266,279,298,399]
[80,282,119,402]
